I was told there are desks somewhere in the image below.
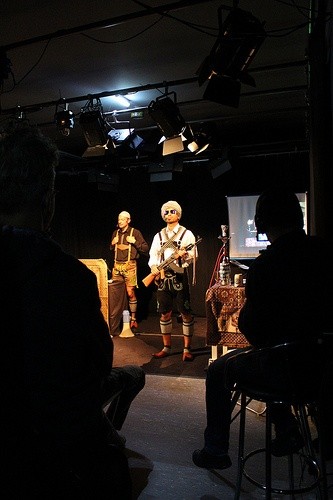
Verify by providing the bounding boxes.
[206,281,251,367]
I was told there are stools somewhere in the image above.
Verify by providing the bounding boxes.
[201,329,333,499]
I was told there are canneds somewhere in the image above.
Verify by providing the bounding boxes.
[234,273,243,287]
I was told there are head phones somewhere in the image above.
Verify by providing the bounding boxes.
[255,216,265,233]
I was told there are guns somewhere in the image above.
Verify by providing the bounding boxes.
[141,237,203,287]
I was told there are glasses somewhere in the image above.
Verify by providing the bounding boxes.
[164,210,177,215]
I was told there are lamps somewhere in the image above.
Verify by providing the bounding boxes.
[54,96,75,136]
[0,49,10,91]
[194,0,268,109]
[2,108,36,144]
[146,83,189,157]
[186,120,215,155]
[79,96,115,158]
[106,113,144,152]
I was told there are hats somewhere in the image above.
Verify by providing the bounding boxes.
[161,201,182,221]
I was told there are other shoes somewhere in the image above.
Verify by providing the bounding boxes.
[152,346,171,358]
[131,320,138,328]
[191,448,231,469]
[272,433,304,457]
[183,347,193,361]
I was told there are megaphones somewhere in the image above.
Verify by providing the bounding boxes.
[119,310,134,337]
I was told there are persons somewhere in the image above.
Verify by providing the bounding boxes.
[0,124,146,444]
[192,187,333,469]
[147,201,199,362]
[109,211,149,334]
